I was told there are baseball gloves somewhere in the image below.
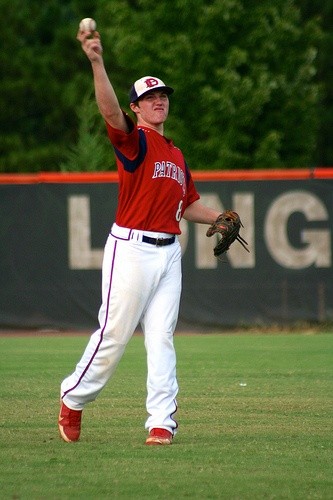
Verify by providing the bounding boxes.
[205,209,250,256]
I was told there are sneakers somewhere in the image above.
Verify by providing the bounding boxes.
[58,397,83,443]
[146,428,173,445]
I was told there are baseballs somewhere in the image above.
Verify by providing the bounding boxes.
[79,18,97,33]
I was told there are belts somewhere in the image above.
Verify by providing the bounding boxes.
[142,235,176,248]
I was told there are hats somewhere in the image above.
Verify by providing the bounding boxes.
[130,76,174,103]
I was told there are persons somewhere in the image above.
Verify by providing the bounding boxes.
[59,28,249,446]
